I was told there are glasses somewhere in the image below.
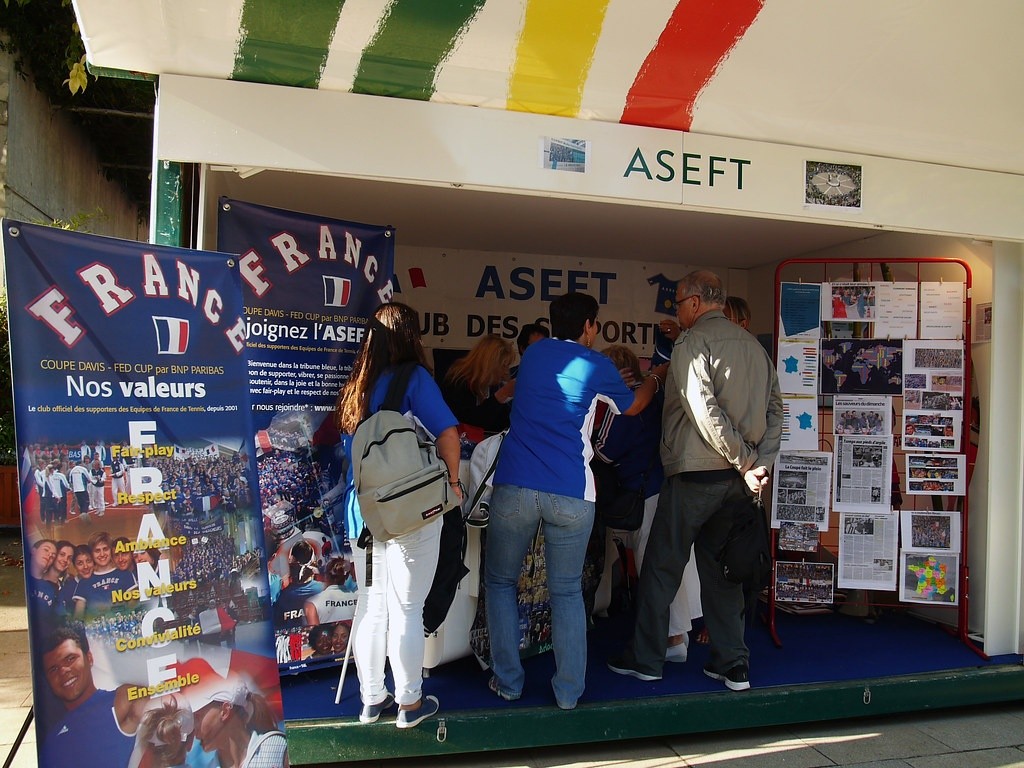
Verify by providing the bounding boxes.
[672,295,700,311]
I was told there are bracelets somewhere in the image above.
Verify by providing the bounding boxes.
[450,479,461,486]
[650,374,664,394]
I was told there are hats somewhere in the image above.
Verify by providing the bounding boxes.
[136,674,254,746]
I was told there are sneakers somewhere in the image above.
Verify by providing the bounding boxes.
[396,695,439,729]
[702,663,751,690]
[359,692,394,723]
[607,657,662,681]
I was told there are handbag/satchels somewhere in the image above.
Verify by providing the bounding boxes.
[602,494,644,531]
[714,485,773,619]
[457,430,542,591]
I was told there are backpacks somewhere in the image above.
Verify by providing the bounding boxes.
[350,358,462,587]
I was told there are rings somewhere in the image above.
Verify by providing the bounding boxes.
[667,328,671,332]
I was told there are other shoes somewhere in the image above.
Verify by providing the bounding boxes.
[555,696,578,709]
[693,625,711,646]
[664,633,689,662]
[41,516,68,531]
[70,500,124,518]
[488,675,521,700]
[864,604,885,625]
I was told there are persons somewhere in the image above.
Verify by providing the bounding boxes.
[608,269,783,690]
[906,415,954,447]
[912,515,950,546]
[845,518,873,535]
[87,609,147,644]
[150,451,248,516]
[777,505,818,551]
[510,296,751,662]
[170,536,251,585]
[836,410,882,435]
[27,531,169,618]
[35,621,149,768]
[778,565,832,601]
[832,286,875,319]
[27,439,127,531]
[141,668,289,768]
[340,302,462,728]
[257,449,358,625]
[275,620,352,663]
[183,595,239,648]
[421,335,518,637]
[485,292,670,709]
